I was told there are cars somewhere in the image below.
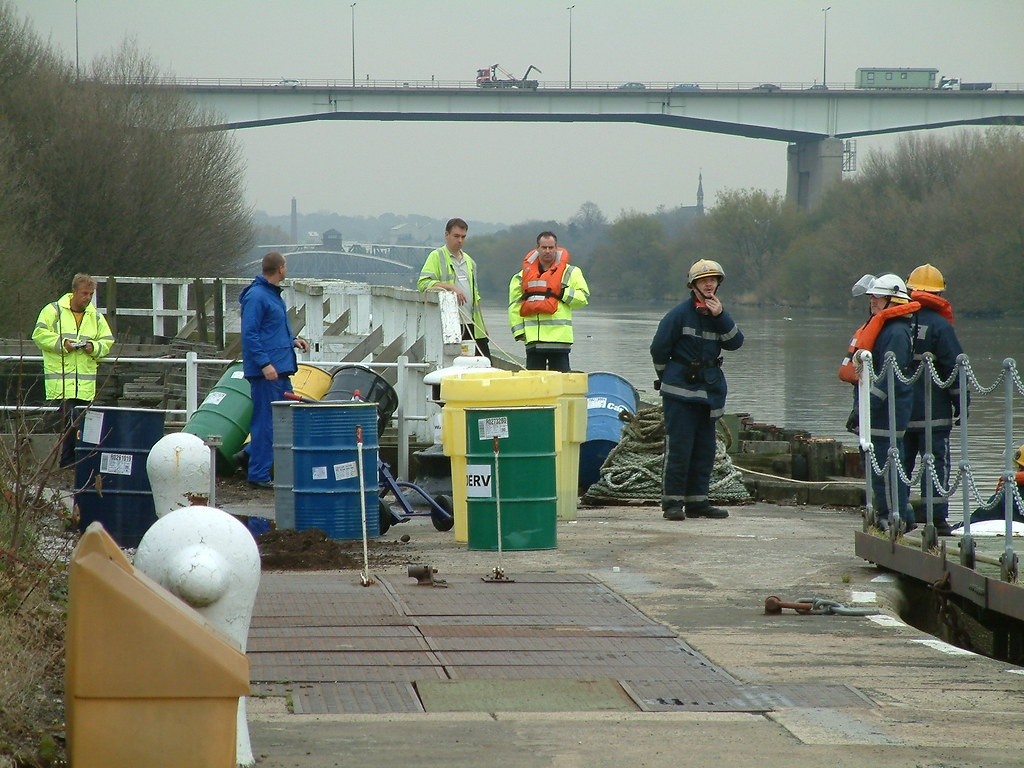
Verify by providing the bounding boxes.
[615,82,645,89]
[752,84,780,89]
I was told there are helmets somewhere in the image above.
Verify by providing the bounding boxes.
[685,259,725,287]
[865,272,912,300]
[1013,443,1024,467]
[906,263,946,297]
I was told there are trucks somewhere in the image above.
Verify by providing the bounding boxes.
[476,65,541,90]
[942,78,992,91]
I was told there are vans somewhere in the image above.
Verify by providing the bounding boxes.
[672,84,700,89]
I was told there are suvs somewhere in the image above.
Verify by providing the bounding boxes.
[805,85,828,90]
[271,80,302,86]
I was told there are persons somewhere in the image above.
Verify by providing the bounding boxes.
[238,252,309,491]
[840,273,918,534]
[416,218,492,407]
[951,445,1024,530]
[649,259,744,521]
[906,261,972,536]
[31,272,115,468]
[508,231,591,373]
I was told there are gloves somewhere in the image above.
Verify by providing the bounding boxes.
[953,405,969,426]
[846,410,859,431]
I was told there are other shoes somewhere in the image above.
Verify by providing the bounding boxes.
[685,504,729,519]
[924,520,955,536]
[663,505,685,520]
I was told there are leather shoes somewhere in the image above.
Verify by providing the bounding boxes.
[248,480,274,490]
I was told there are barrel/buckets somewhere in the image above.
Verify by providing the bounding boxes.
[238,366,332,488]
[579,371,641,489]
[463,405,558,551]
[182,361,252,477]
[290,402,381,540]
[71,408,167,548]
[271,399,352,532]
[319,364,400,438]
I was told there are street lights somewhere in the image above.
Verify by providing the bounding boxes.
[75,0,78,77]
[350,3,355,87]
[822,6,830,85]
